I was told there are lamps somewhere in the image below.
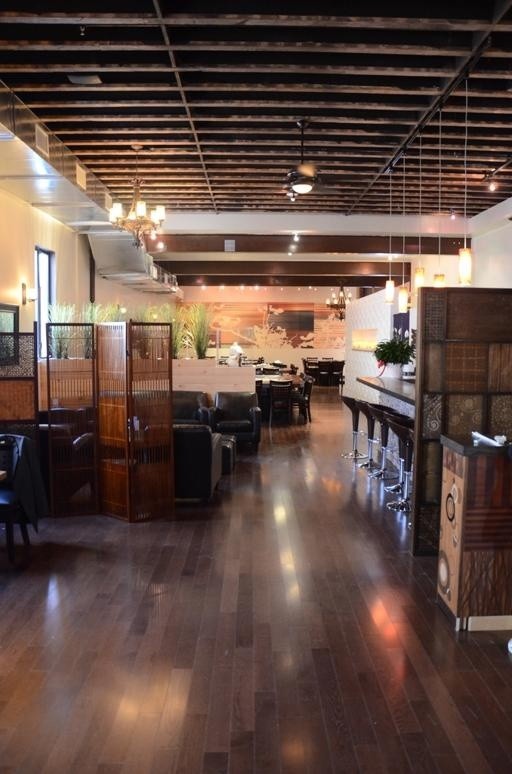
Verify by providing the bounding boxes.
[21,282,39,306]
[482,172,497,194]
[287,114,318,195]
[387,74,473,314]
[104,143,168,249]
[324,279,353,320]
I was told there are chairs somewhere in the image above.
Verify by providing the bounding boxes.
[1,434,38,563]
[302,356,345,387]
[256,360,317,428]
[173,356,265,367]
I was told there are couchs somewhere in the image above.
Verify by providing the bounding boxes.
[38,390,237,504]
[208,391,262,456]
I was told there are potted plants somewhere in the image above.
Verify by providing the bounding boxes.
[373,333,415,380]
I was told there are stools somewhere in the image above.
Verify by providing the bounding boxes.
[356,398,380,474]
[386,420,415,514]
[338,394,368,461]
[385,414,411,493]
[366,402,400,482]
